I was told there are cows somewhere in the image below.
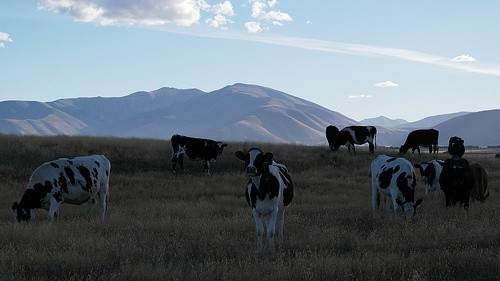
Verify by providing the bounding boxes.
[399,129,439,157]
[439,155,490,208]
[370,155,423,220]
[170,135,228,177]
[235,148,294,250]
[415,159,445,195]
[11,154,111,225]
[448,136,466,158]
[326,125,377,155]
